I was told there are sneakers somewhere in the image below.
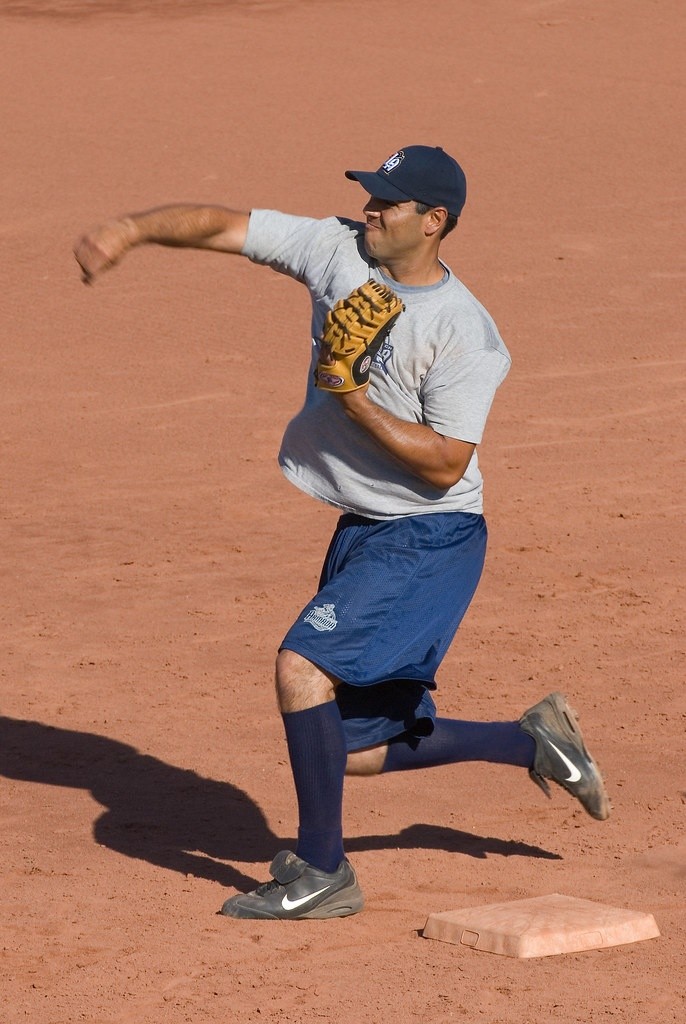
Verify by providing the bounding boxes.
[516,690,610,821]
[220,849,363,920]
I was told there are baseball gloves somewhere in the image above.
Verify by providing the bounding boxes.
[310,276,406,394]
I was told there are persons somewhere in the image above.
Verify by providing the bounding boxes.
[69,146,610,921]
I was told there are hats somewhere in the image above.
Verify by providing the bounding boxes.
[344,145,466,217]
